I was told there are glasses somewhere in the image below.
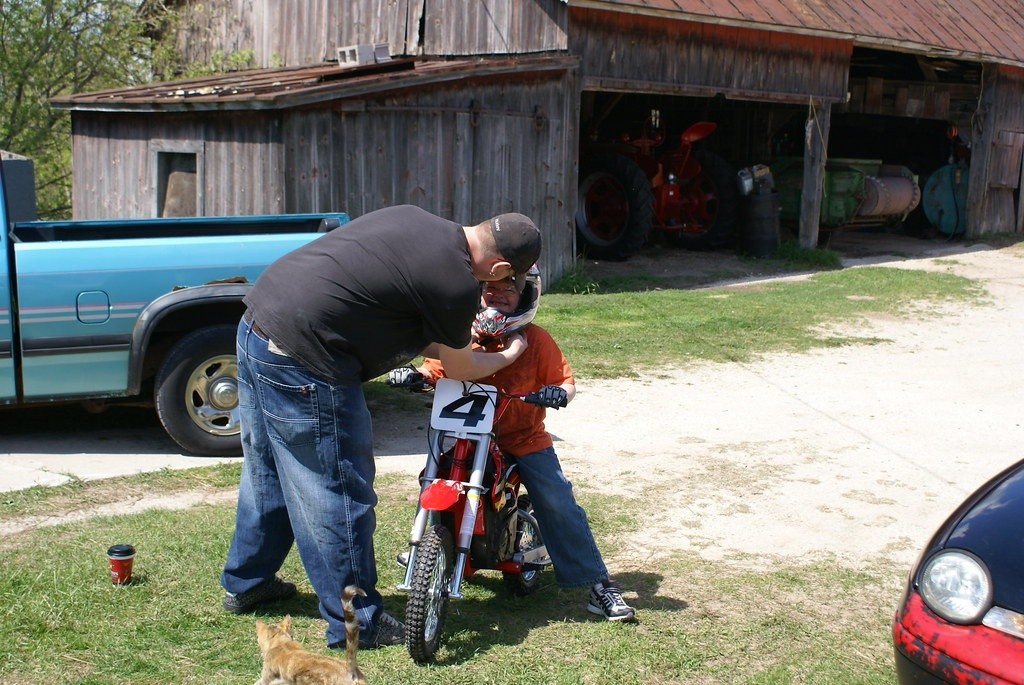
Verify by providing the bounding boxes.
[505,266,515,284]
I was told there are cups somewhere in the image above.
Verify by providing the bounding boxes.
[106,544,136,586]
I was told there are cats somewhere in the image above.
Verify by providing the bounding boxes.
[253,585,370,685]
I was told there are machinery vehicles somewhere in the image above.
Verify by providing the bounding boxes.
[579,119,744,263]
[765,156,921,245]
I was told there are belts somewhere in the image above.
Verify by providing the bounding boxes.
[244,308,269,340]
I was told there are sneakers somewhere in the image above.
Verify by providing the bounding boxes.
[587,580,636,621]
[222,574,297,612]
[397,552,410,568]
[376,612,406,647]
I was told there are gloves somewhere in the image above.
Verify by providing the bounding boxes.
[538,384,567,408]
[388,365,417,386]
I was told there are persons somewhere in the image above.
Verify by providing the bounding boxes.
[397,261,635,623]
[219,204,543,647]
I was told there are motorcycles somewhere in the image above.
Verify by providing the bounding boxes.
[385,371,552,661]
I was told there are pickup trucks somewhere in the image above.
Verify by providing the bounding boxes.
[0,177,349,460]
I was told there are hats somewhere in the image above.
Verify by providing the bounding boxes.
[491,212,543,294]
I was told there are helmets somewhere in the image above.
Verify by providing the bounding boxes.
[472,262,542,336]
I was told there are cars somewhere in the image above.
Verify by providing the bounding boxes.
[892,459,1023,685]
